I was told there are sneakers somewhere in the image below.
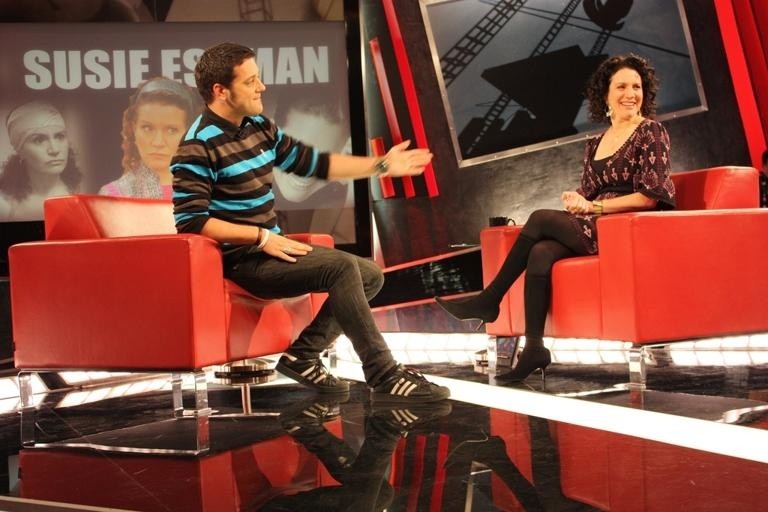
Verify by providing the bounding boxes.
[363,398,451,435]
[370,364,450,401]
[276,353,350,394]
[278,393,350,429]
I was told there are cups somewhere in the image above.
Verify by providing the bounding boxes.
[489,216,516,227]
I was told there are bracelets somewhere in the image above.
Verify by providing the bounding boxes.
[253,227,264,245]
[257,229,270,249]
[592,199,604,214]
[373,157,390,178]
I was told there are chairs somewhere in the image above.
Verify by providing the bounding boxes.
[8,195,335,457]
[480,167,768,390]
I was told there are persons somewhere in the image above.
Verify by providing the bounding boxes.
[430,50,680,383]
[253,395,453,512]
[441,395,576,510]
[94,73,206,205]
[245,65,356,242]
[168,35,454,405]
[1,100,85,225]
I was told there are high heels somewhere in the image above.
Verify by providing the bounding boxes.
[433,296,498,331]
[442,428,507,470]
[494,348,552,383]
